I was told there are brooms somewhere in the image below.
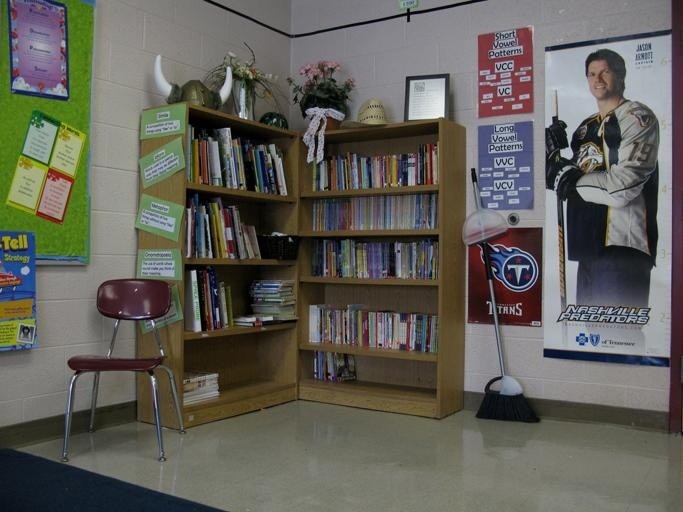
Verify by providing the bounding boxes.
[470,166,544,424]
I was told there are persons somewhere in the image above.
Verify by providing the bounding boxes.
[545,46,660,354]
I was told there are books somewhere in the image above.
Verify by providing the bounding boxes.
[309,304,439,383]
[184,369,220,405]
[312,141,439,280]
[184,123,288,258]
[184,266,299,334]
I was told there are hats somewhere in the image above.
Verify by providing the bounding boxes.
[339,96,393,129]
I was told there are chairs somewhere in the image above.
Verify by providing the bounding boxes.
[61,279,187,464]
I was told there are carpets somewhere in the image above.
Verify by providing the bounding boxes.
[0,447,230,512]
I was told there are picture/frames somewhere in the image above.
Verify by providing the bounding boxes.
[404,74,450,120]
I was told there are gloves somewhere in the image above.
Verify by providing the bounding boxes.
[546,156,585,202]
[545,120,569,158]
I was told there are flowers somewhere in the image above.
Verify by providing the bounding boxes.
[288,60,356,119]
